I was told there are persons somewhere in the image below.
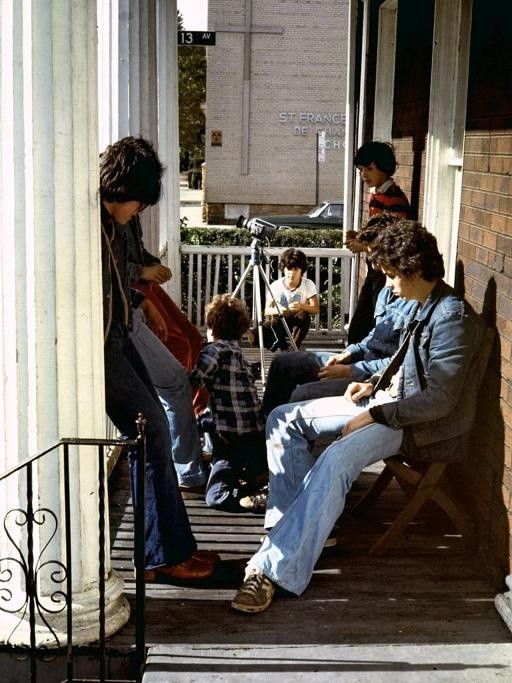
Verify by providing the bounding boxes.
[119,290,213,493]
[266,249,320,352]
[229,222,476,614]
[124,215,214,419]
[186,294,265,513]
[350,142,413,342]
[239,213,422,511]
[94,139,220,580]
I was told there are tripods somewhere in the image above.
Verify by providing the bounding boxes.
[232,247,300,394]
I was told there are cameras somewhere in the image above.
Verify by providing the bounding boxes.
[237,217,278,246]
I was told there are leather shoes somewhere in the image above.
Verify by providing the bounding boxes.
[180,482,207,501]
[191,544,222,567]
[141,556,219,584]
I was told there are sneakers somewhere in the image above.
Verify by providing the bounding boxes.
[230,574,276,614]
[238,483,270,513]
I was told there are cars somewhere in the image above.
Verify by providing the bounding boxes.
[251,201,344,233]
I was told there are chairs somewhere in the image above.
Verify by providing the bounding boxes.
[351,327,495,556]
